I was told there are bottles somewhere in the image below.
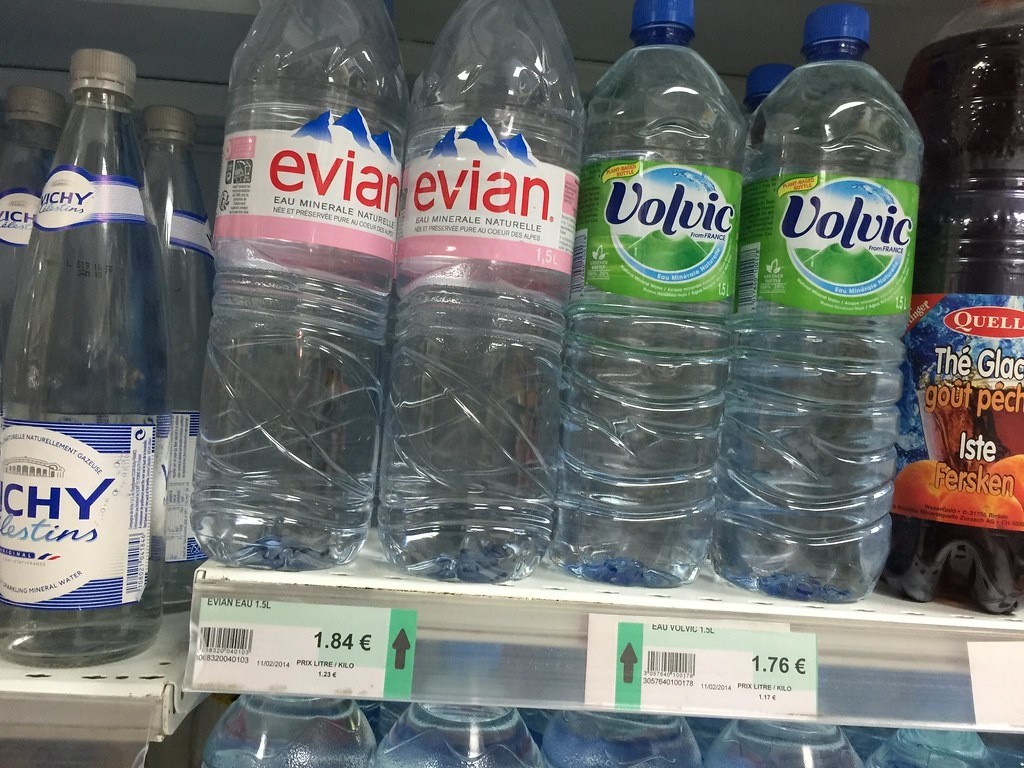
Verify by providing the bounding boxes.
[0,0,1024,768]
